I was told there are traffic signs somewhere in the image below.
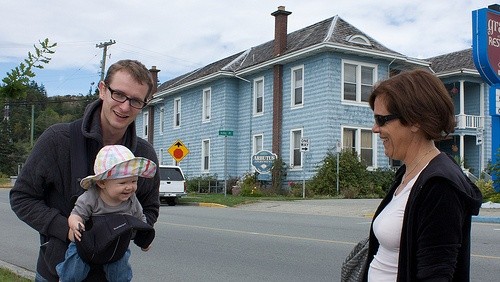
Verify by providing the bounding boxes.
[299,138,310,152]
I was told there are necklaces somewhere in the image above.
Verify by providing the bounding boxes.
[401,147,437,184]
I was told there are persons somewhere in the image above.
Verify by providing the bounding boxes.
[362,69,483,282]
[9,59,160,282]
[56,144,157,282]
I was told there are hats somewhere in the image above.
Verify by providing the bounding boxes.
[80,145,157,190]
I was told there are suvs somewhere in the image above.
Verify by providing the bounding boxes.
[158,164,188,206]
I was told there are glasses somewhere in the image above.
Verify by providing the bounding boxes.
[374,113,403,128]
[107,86,148,109]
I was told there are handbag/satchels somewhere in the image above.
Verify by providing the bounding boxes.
[341,235,369,282]
